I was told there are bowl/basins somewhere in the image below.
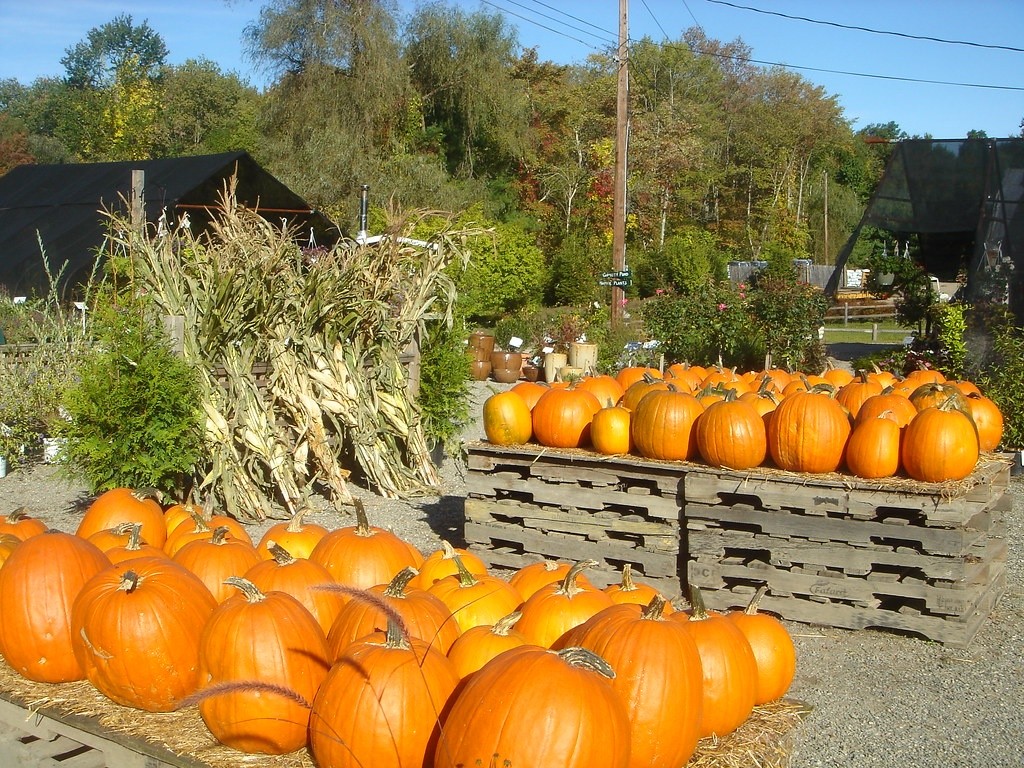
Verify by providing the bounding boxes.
[493,369,520,383]
[470,360,491,381]
[490,352,522,369]
[559,366,582,383]
[522,367,545,382]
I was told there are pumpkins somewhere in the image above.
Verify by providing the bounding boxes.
[0,485,795,768]
[482,360,1004,482]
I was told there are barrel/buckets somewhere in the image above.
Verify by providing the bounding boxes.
[546,353,567,384]
[570,342,597,375]
[468,335,494,362]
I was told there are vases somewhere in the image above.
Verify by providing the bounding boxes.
[493,369,521,383]
[468,334,495,362]
[556,367,583,382]
[469,361,492,381]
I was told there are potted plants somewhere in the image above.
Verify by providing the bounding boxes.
[545,316,585,384]
[569,309,608,372]
[0,348,45,478]
[520,303,556,381]
[491,315,532,370]
[20,230,110,464]
